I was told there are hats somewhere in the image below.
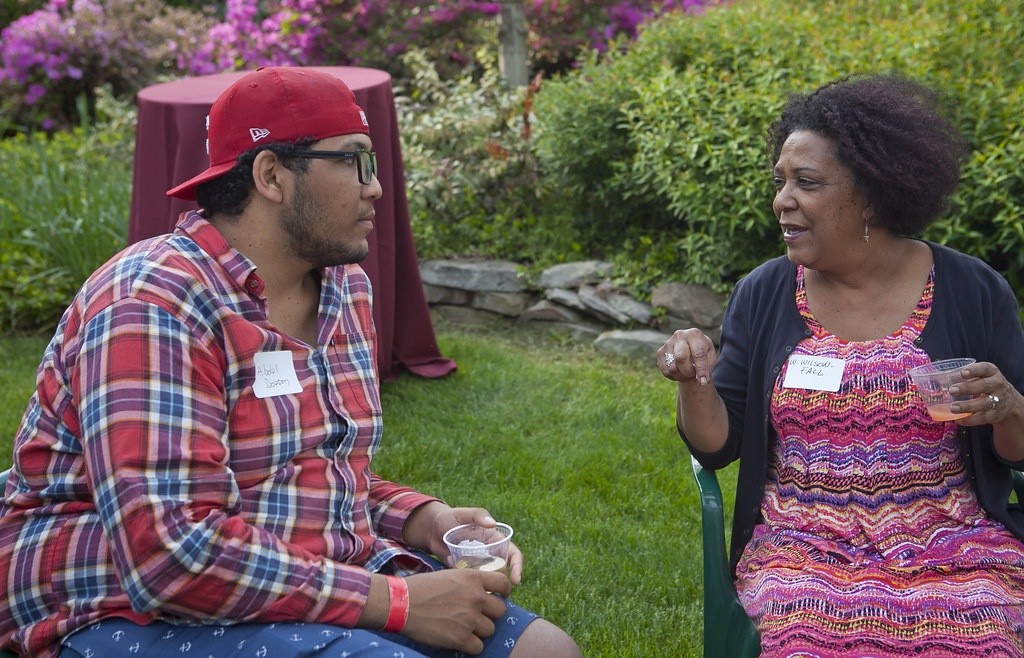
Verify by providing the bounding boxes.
[166,66,370,201]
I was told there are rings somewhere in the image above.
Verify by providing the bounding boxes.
[664,352,675,366]
[989,394,999,409]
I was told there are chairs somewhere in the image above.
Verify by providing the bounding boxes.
[690,456,1024,658]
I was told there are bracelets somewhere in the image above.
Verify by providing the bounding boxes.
[381,575,410,632]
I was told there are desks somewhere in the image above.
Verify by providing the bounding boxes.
[128,66,460,388]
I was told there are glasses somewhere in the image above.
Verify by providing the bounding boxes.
[283,149,378,186]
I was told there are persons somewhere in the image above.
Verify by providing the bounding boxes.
[656,74,1024,658]
[0,66,584,658]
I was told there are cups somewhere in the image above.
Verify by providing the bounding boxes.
[442,522,514,595]
[908,358,976,421]
